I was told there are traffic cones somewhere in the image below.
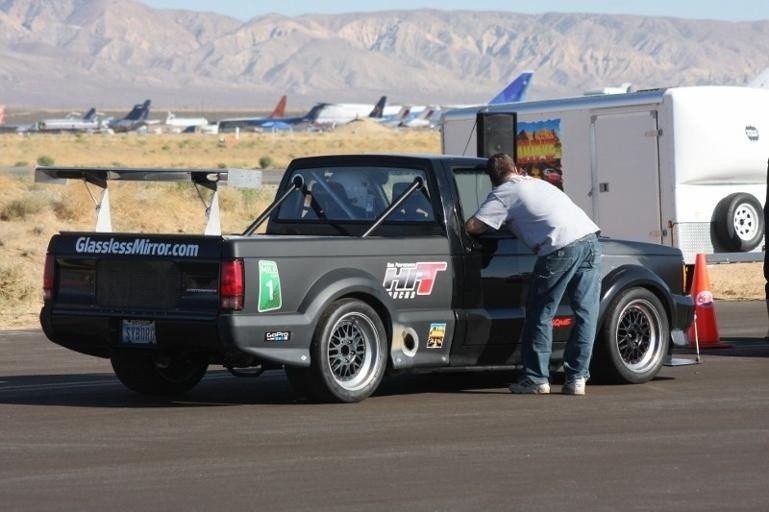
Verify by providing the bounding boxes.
[677,243,742,352]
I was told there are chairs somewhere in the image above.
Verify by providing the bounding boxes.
[304,182,365,219]
[376,182,425,218]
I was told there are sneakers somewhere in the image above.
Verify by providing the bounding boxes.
[562,375,587,396]
[507,376,552,395]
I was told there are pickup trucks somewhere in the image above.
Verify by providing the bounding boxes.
[28,150,700,409]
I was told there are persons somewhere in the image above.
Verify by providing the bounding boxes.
[464,152,601,396]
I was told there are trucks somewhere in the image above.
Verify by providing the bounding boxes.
[438,79,768,280]
